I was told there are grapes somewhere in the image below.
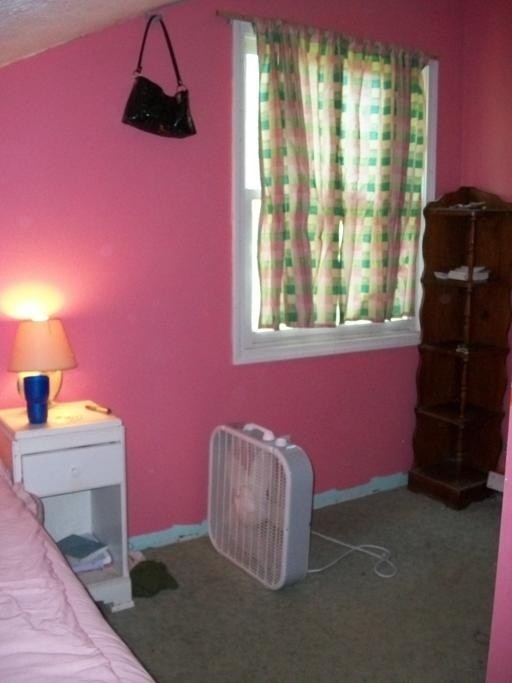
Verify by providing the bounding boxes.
[0,400,135,613]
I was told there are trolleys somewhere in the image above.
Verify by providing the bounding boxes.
[206,424,314,589]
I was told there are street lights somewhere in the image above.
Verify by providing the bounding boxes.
[122,74,198,139]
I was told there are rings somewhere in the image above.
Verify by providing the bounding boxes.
[85,406,112,415]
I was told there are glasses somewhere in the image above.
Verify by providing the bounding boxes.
[5,319,78,407]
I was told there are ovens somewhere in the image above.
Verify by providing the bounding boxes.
[0,463,154,683]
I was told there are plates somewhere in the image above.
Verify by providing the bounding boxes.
[408,185,512,511]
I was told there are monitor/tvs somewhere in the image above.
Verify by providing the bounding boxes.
[432,264,489,280]
[54,533,109,561]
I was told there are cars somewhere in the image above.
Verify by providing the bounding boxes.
[23,375,50,424]
